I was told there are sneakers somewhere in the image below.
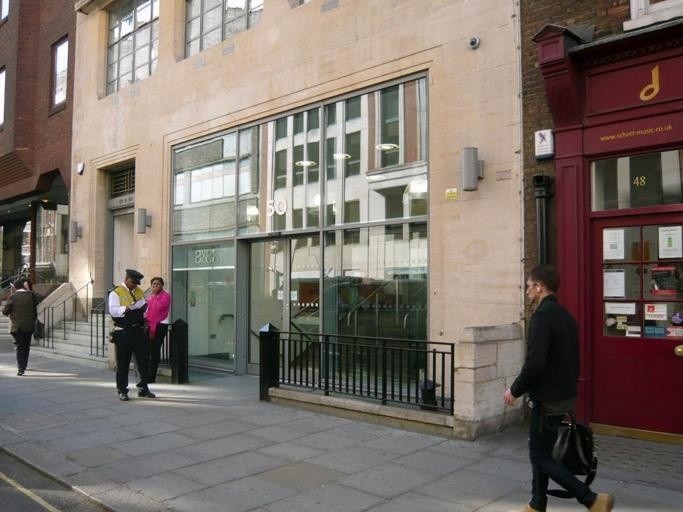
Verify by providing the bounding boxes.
[147,378,156,384]
[17,370,24,375]
[119,392,129,401]
[138,391,155,398]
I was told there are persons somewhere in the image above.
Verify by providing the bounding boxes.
[136,276,170,387]
[108,269,156,401]
[11,280,35,349]
[503,265,615,512]
[2,279,35,375]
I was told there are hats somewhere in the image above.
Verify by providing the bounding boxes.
[125,269,144,285]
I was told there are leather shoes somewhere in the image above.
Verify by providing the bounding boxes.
[505,502,545,512]
[588,492,615,512]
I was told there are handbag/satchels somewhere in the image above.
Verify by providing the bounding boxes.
[33,320,44,340]
[550,412,594,476]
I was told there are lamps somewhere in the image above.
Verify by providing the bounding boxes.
[133,208,151,234]
[462,146,485,191]
[70,222,83,243]
